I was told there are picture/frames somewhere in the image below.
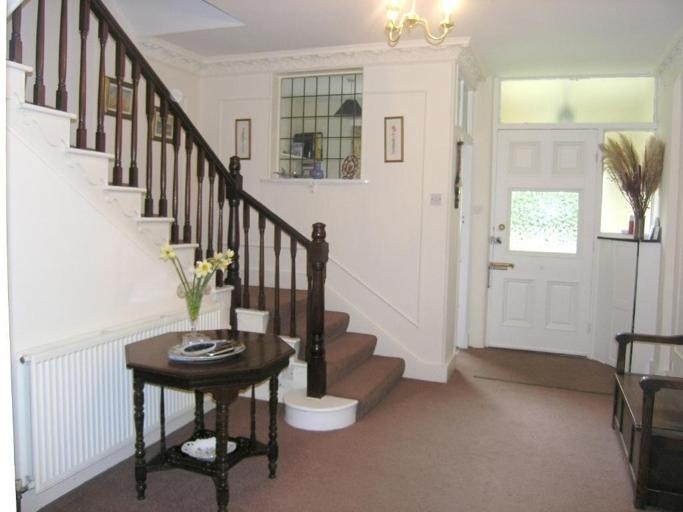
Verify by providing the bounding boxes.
[383,115,404,162]
[235,118,251,161]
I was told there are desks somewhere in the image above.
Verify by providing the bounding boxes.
[124,329,296,511]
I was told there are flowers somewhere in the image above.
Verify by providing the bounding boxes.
[159,244,238,322]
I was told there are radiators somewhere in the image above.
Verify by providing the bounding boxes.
[16,302,221,512]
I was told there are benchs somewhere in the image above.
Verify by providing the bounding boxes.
[612,318,681,508]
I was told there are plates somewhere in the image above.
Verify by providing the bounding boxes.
[180,436,237,462]
[167,339,246,365]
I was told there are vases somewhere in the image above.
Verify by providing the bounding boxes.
[175,281,215,343]
